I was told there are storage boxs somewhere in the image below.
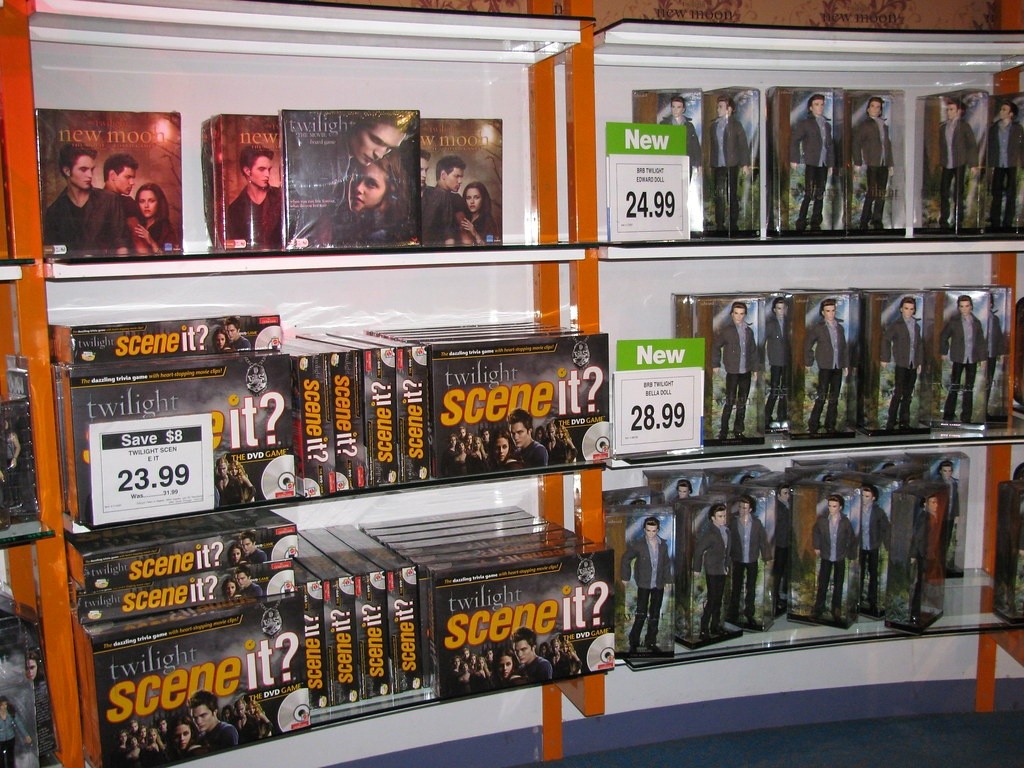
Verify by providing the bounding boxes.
[4,86,1024,767]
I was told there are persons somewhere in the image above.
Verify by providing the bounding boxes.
[45,141,177,256]
[448,622,583,700]
[226,143,283,246]
[659,94,1024,236]
[212,317,251,352]
[438,408,577,477]
[711,295,1006,441]
[114,691,279,767]
[618,461,960,652]
[0,652,56,768]
[306,113,418,245]
[213,456,257,505]
[221,529,266,598]
[419,148,497,245]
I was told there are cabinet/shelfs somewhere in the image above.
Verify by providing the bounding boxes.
[0,0,1024,767]
